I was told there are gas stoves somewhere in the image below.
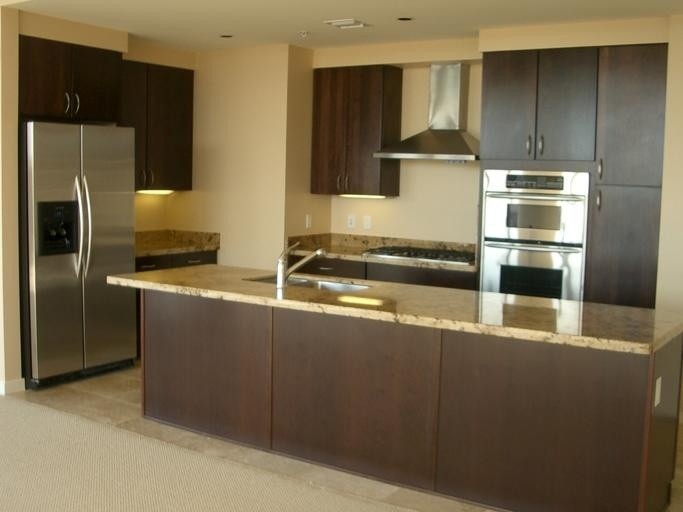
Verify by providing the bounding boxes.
[363,242,472,266]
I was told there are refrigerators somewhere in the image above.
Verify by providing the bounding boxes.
[19,119,138,388]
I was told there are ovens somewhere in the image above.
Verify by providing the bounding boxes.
[478,168,590,303]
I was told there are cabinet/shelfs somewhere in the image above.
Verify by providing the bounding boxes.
[288,256,367,282]
[310,68,400,196]
[121,58,194,191]
[588,43,669,306]
[478,49,598,161]
[16,31,124,124]
[136,250,217,281]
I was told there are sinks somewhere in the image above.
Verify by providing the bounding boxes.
[243,274,311,287]
[293,280,372,293]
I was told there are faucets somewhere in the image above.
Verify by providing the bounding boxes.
[276,241,322,289]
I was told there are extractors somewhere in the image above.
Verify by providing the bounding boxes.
[372,62,479,164]
